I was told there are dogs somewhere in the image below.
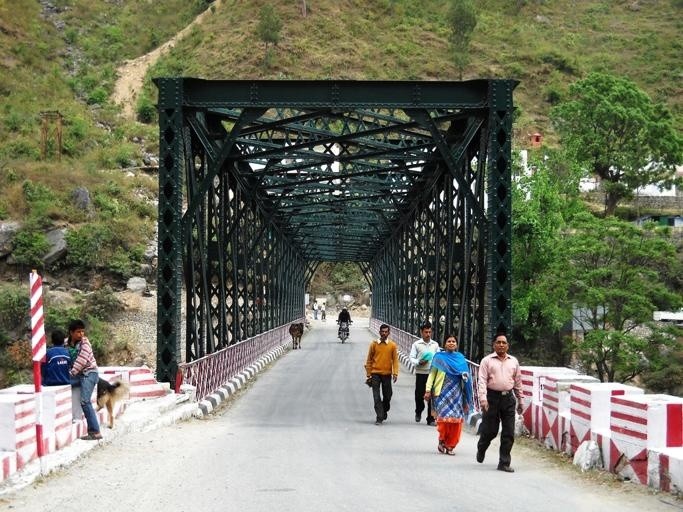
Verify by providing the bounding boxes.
[95,377,128,429]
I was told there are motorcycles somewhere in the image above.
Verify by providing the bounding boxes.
[335,319,352,344]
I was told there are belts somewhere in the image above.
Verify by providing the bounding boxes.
[487,388,511,395]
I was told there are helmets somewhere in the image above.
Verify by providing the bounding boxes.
[342,307,347,313]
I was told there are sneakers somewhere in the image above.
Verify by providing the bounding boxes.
[447,447,457,455]
[375,420,383,426]
[382,418,388,423]
[80,430,103,441]
[437,444,445,454]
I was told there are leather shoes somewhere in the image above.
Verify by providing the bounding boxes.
[415,413,421,421]
[427,420,437,426]
[477,450,485,463]
[497,461,514,472]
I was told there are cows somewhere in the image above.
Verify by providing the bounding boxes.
[289,323,303,350]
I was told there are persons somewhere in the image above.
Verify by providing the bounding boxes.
[338,307,350,336]
[409,321,441,426]
[423,334,473,455]
[476,334,524,472]
[40,330,79,387]
[320,302,325,319]
[364,323,399,424]
[312,300,318,319]
[63,319,102,440]
